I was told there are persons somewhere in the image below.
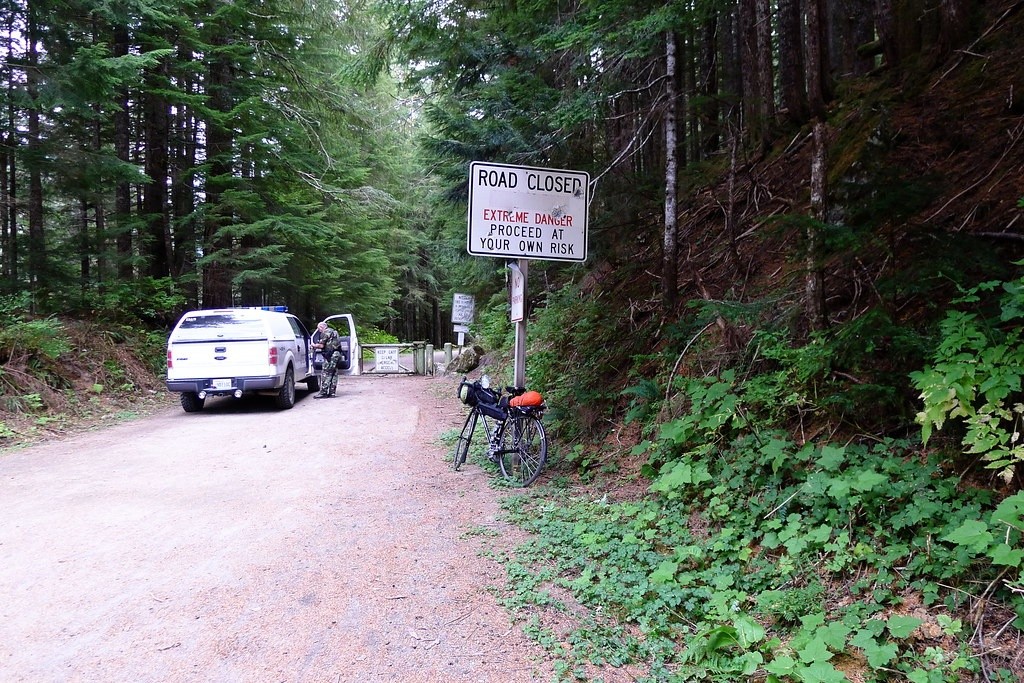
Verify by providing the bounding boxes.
[312,322,343,399]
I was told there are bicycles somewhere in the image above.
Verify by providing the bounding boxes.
[453,376,546,488]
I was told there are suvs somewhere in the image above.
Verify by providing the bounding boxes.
[166,305,360,413]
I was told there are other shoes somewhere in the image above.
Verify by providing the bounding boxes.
[327,394,335,398]
[314,393,327,398]
